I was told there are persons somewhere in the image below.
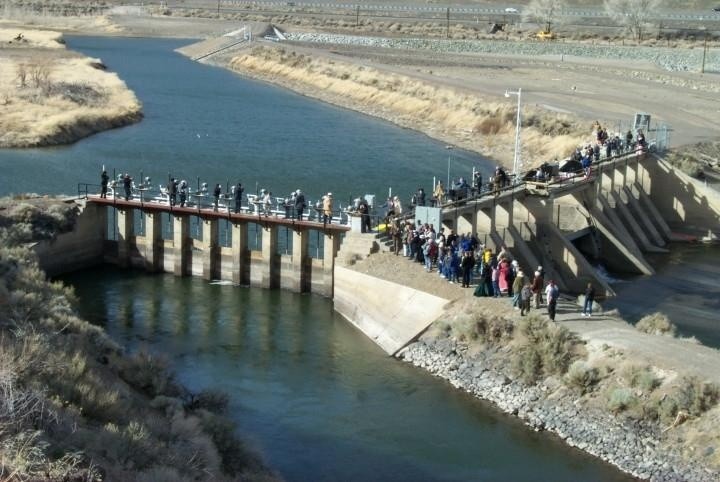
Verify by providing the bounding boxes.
[490,165,506,197]
[383,195,402,220]
[593,121,645,158]
[532,144,594,182]
[100,171,305,221]
[356,196,372,233]
[411,172,482,207]
[581,282,596,318]
[390,217,481,288]
[473,246,560,321]
[323,192,334,224]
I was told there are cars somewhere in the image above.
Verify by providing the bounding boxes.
[505,7,518,13]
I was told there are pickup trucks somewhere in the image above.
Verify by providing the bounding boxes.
[537,30,553,39]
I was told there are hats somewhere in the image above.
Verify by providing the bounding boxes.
[517,271,525,277]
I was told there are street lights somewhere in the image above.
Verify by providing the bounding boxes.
[445,145,454,193]
[503,88,522,178]
